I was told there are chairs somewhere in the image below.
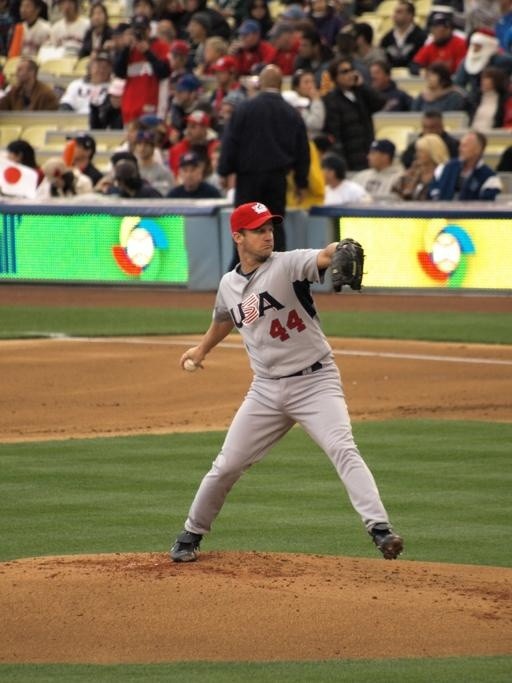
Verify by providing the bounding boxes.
[0,0,511,154]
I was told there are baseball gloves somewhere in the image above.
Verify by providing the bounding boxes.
[330,239,364,292]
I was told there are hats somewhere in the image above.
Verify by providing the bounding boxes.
[175,74,200,91]
[238,75,261,91]
[66,133,95,152]
[178,150,200,168]
[134,129,154,141]
[234,20,259,33]
[131,13,148,26]
[230,202,284,232]
[279,4,302,20]
[139,115,164,129]
[268,22,291,36]
[370,140,394,153]
[321,156,343,169]
[222,89,245,108]
[312,136,333,152]
[112,159,139,180]
[429,13,449,24]
[183,110,211,126]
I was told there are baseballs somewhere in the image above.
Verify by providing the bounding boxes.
[183,358,199,372]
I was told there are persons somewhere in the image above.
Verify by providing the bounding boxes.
[0,0,512,206]
[167,199,407,563]
[214,61,311,272]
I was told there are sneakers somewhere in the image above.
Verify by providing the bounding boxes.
[169,531,203,562]
[368,522,403,560]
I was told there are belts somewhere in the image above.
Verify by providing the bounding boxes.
[280,361,322,378]
